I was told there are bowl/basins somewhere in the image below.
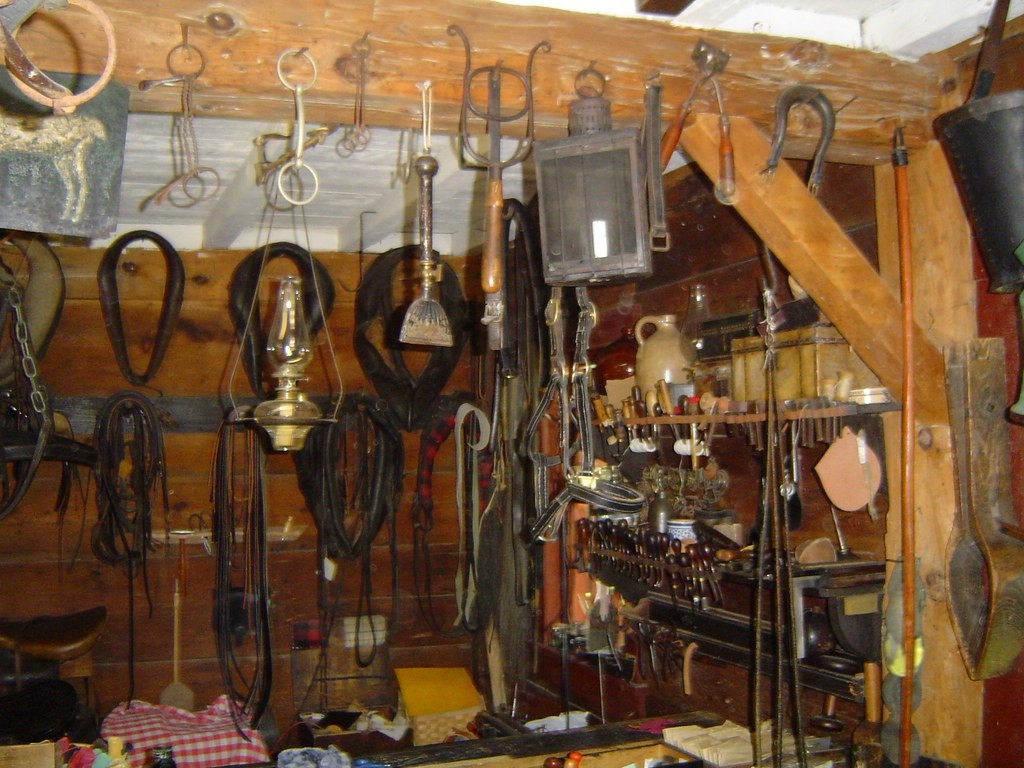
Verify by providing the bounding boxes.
[852,387,891,405]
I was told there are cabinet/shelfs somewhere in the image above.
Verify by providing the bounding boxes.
[525,270,897,768]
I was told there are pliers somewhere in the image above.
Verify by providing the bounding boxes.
[636,620,657,680]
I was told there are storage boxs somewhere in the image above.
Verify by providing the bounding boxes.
[0,744,63,768]
[731,326,882,401]
[393,666,487,747]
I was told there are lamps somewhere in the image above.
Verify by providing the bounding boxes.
[228,157,342,449]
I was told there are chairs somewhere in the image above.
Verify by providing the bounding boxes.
[0,606,107,694]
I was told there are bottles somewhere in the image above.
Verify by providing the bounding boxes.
[563,751,582,768]
[648,491,674,535]
[635,314,698,402]
[592,327,639,395]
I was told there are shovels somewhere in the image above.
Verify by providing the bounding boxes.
[399,147,454,348]
[157,576,195,711]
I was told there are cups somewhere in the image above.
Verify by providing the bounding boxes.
[667,519,698,543]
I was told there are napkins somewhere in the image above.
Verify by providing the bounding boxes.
[663,722,796,764]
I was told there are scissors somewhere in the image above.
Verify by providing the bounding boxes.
[188,510,212,557]
